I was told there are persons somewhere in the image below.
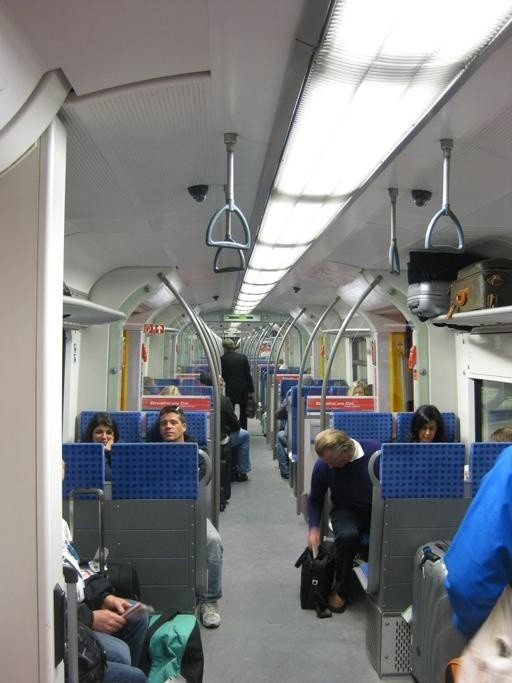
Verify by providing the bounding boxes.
[464,427,512,478]
[62,457,149,683]
[144,338,369,480]
[408,405,445,443]
[309,429,381,613]
[444,445,512,683]
[73,406,224,630]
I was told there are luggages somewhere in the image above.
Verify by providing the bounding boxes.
[412,539,468,683]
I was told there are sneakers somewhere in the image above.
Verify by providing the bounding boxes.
[200,603,222,628]
[231,473,248,482]
[281,474,289,478]
[328,592,346,613]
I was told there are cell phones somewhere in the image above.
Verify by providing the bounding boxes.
[121,602,141,617]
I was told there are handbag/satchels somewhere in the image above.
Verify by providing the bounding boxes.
[444,656,485,683]
[146,608,204,683]
[294,541,335,616]
[246,393,255,419]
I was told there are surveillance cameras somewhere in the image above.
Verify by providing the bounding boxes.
[411,190,432,207]
[213,296,218,301]
[187,185,208,202]
[292,287,300,294]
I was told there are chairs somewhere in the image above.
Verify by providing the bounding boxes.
[64,361,216,618]
[322,408,460,554]
[253,360,352,496]
[352,440,512,677]
